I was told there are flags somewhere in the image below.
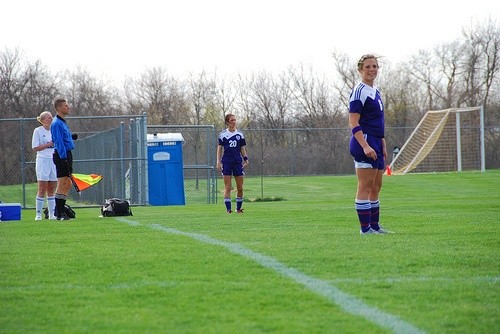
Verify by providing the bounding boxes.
[68,171,103,192]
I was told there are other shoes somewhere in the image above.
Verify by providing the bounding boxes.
[227,209,233,214]
[235,208,244,214]
[35,216,42,220]
[360,227,385,235]
[57,215,69,220]
[376,226,395,233]
[49,216,57,220]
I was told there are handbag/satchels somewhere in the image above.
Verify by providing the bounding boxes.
[45,204,75,219]
[101,198,131,217]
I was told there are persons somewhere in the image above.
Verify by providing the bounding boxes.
[31,111,57,221]
[348,54,394,236]
[216,114,249,215]
[50,99,75,220]
